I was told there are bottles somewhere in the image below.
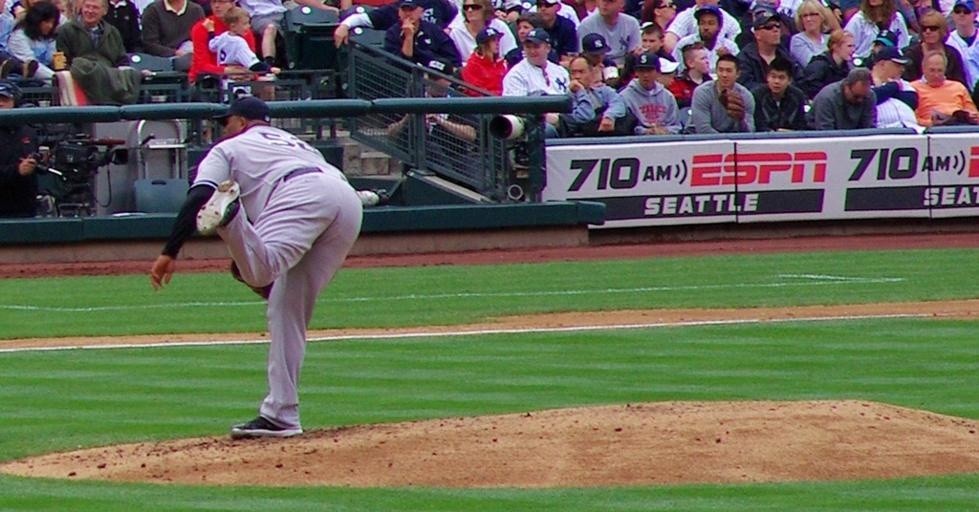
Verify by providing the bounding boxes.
[399,0,425,9]
[0,80,18,99]
[953,0,976,13]
[536,0,561,5]
[522,26,554,47]
[634,52,663,71]
[474,26,506,45]
[873,47,912,68]
[424,56,456,78]
[693,3,725,26]
[581,32,611,57]
[753,13,782,32]
[209,94,275,124]
[873,28,900,50]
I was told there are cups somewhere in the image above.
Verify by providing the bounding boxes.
[848,85,872,100]
[656,2,676,11]
[462,3,488,12]
[535,2,558,9]
[920,23,942,32]
[756,24,781,31]
[954,8,973,15]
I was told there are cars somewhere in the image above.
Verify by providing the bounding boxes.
[602,66,620,81]
[53,51,67,70]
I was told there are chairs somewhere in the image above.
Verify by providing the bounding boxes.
[1,0,393,144]
[228,412,305,440]
[194,178,245,238]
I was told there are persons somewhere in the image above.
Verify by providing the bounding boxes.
[383,0,979,191]
[0,0,291,221]
[151,95,364,440]
[283,1,457,48]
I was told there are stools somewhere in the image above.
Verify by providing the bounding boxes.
[280,165,325,182]
